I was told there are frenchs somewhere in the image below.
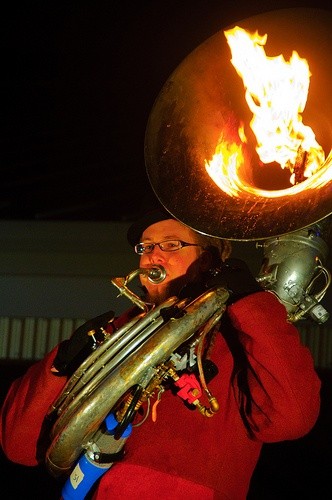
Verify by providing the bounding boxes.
[40,0,332,500]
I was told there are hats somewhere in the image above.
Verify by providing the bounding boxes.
[127,206,197,245]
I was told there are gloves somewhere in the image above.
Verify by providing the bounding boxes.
[53,312,113,374]
[181,260,259,304]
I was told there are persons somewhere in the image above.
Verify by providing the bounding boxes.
[0,207,321,499]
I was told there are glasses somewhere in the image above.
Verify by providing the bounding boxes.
[135,240,209,256]
